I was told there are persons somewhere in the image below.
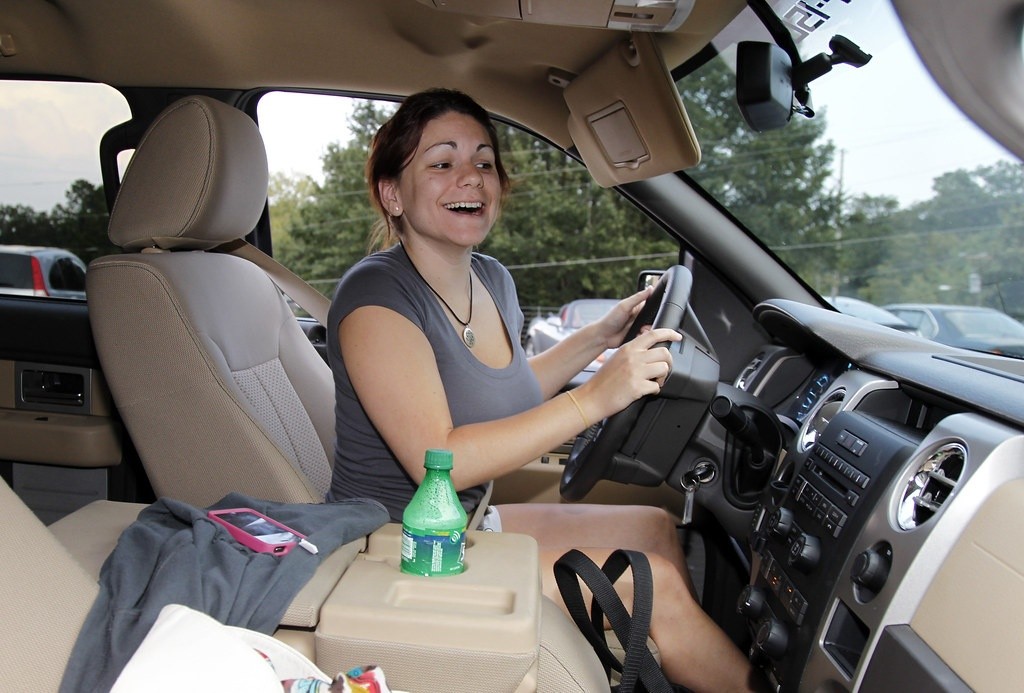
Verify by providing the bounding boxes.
[326,89,769,693]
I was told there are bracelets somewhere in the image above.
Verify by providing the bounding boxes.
[566,391,589,429]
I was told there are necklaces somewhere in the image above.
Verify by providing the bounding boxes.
[400,240,475,348]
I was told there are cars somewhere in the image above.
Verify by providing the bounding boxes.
[881,302,1024,359]
[821,295,923,340]
[1,243,87,301]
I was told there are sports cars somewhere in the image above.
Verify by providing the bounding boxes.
[524,298,630,394]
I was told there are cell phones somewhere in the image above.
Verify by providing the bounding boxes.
[207,508,307,555]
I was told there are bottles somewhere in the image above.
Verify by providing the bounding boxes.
[398,447,469,580]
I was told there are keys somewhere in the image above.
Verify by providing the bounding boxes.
[679,463,714,490]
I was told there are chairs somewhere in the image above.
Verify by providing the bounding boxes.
[85,96,336,507]
[0,476,100,693]
